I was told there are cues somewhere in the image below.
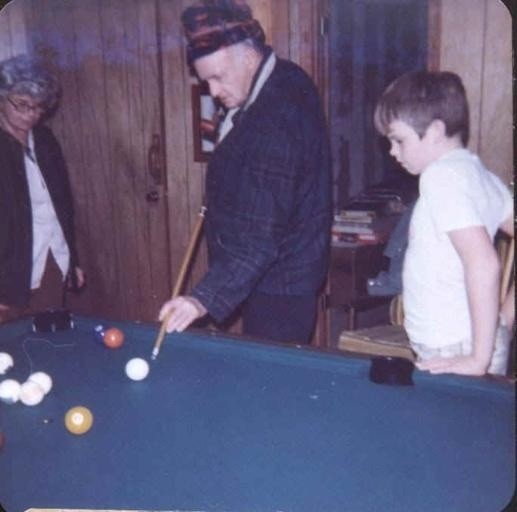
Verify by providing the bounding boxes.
[151,198,208,361]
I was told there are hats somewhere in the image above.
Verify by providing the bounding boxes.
[180,0,266,60]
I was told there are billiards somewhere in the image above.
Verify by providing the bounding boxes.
[64,406,92,435]
[125,358,149,380]
[92,323,124,349]
[0,352,52,406]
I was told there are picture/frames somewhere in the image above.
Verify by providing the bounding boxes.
[192,88,230,162]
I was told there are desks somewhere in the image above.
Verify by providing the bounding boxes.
[321,238,420,334]
[0,293,516,511]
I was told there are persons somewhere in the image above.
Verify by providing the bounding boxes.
[373,71,515,377]
[158,0,333,345]
[0,52,85,324]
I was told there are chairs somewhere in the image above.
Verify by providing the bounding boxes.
[336,226,515,361]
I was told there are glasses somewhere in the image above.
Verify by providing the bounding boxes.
[6,95,54,114]
